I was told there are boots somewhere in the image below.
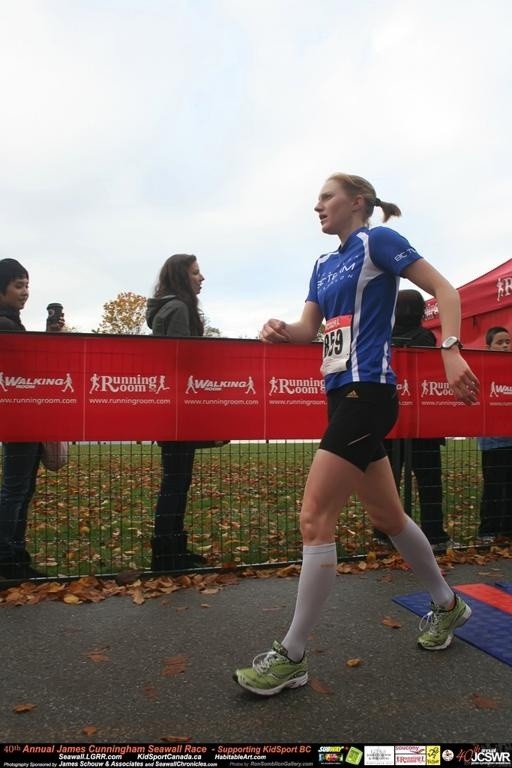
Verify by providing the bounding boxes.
[150,530,207,571]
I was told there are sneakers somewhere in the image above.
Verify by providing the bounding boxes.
[233,639,308,696]
[417,592,472,651]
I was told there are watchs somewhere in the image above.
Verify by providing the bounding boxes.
[439,335,462,351]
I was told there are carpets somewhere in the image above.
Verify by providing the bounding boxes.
[386,577,512,668]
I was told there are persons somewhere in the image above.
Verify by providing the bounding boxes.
[146,253,228,569]
[234,173,480,699]
[478,326,512,540]
[373,289,462,550]
[0,258,64,582]
